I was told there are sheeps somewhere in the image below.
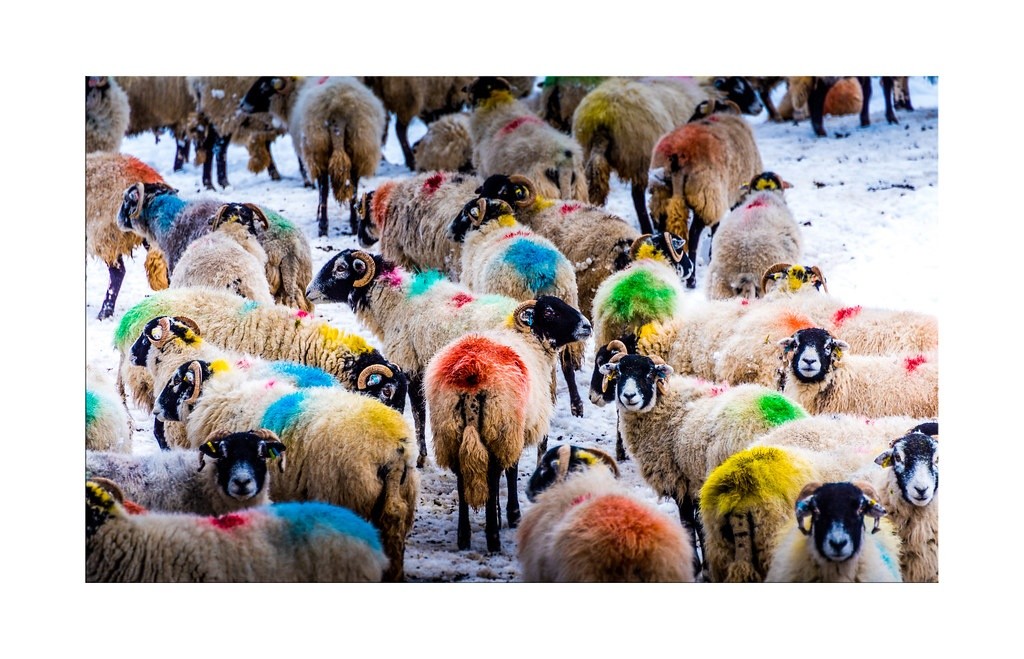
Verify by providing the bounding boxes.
[86,76,939,583]
